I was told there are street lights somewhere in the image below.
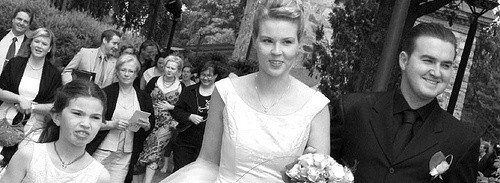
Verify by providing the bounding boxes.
[164,0,183,56]
[446,0,500,116]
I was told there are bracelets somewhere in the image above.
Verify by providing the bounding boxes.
[31,103,36,113]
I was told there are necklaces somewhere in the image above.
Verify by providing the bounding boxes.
[255,72,292,113]
[54,141,86,168]
[29,58,44,71]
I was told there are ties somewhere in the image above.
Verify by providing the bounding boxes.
[92,55,105,84]
[3,38,17,68]
[388,110,419,163]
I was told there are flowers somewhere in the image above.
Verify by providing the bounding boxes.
[428,151,453,181]
[281,153,354,183]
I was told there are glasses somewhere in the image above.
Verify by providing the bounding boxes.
[200,73,214,79]
[118,68,137,74]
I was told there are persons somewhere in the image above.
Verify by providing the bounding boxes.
[85,53,154,182]
[61,30,123,89]
[0,27,64,171]
[134,55,187,183]
[170,60,221,173]
[330,22,481,183]
[1,78,112,183]
[159,0,333,182]
[477,139,500,183]
[159,64,197,174]
[115,39,204,93]
[0,6,33,79]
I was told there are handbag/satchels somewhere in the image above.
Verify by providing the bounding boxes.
[0,104,26,146]
